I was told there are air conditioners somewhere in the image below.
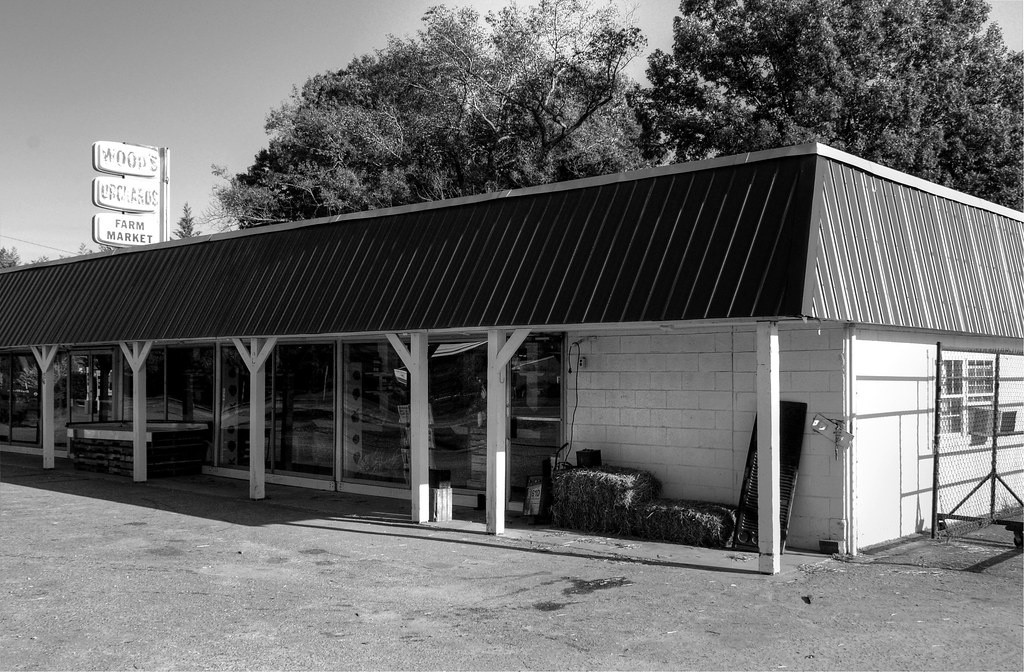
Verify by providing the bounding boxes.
[969,406,1017,437]
